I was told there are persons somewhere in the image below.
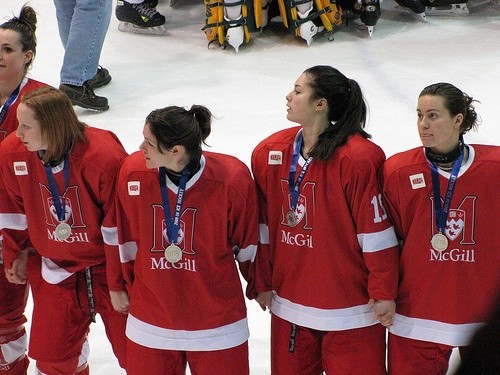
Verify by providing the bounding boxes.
[104,105,274,375]
[0,4,51,375]
[336,0,380,38]
[0,85,129,375]
[201,0,343,53]
[383,83,500,375]
[252,63,400,375]
[116,0,166,35]
[395,0,471,23]
[55,0,112,112]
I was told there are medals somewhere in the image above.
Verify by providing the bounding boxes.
[286,210,297,228]
[431,232,449,252]
[165,244,181,263]
[55,221,75,240]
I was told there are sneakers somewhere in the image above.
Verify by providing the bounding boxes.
[394,0,427,23]
[59,83,108,111]
[84,64,112,88]
[361,0,380,37]
[116,0,167,34]
[426,0,470,16]
